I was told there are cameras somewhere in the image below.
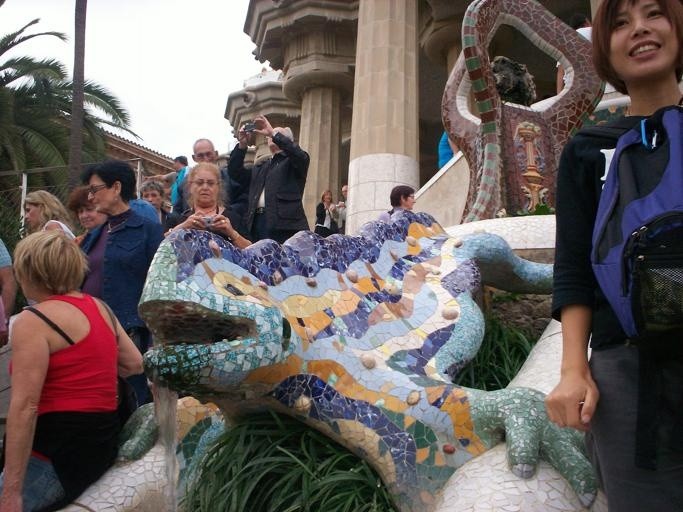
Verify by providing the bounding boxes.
[243,122,258,132]
[200,217,214,228]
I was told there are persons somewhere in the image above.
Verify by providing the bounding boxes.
[388,187,415,213]
[1,230,145,510]
[168,155,187,209]
[80,161,163,348]
[438,127,457,170]
[328,184,346,233]
[138,180,172,224]
[177,141,217,207]
[70,185,103,244]
[0,236,18,343]
[313,190,333,239]
[544,1,682,511]
[164,162,254,249]
[22,191,75,235]
[226,114,310,242]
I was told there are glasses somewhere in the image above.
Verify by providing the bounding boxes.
[87,184,108,194]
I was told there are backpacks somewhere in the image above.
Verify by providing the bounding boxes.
[592,106,683,471]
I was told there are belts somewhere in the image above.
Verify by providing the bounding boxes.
[256,207,264,215]
